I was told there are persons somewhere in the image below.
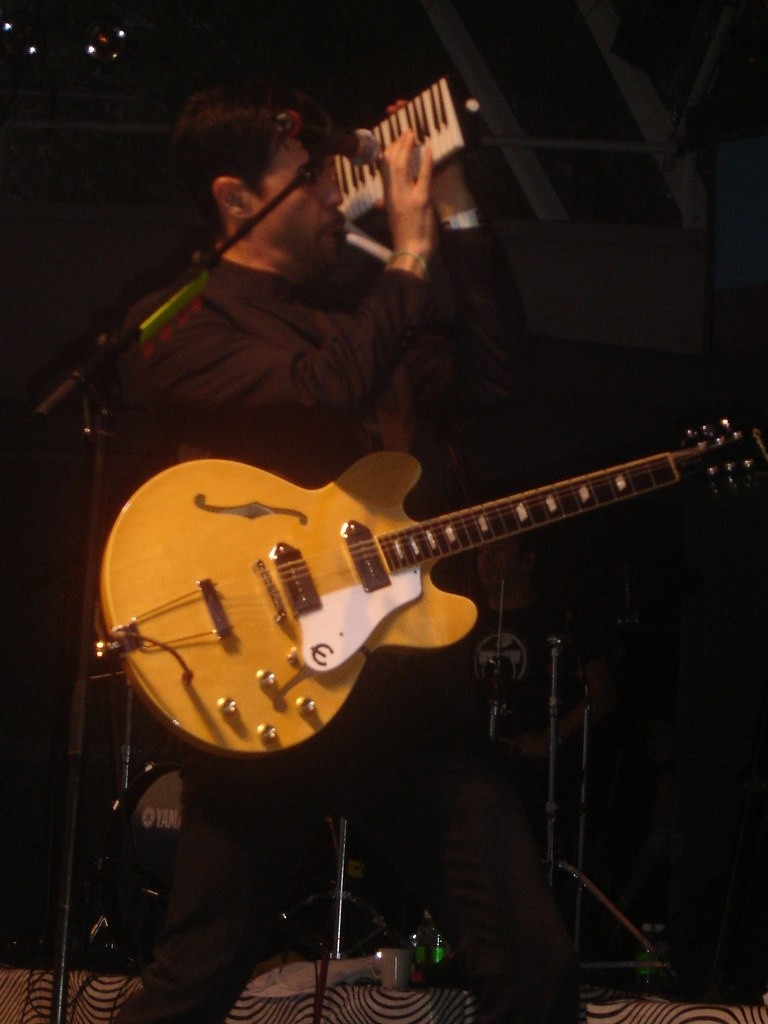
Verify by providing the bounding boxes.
[106,71,583,1024]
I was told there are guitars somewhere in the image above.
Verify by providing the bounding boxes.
[98,420,768,758]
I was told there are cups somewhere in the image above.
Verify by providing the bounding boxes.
[371,950,410,988]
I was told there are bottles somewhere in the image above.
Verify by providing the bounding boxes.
[430,918,448,983]
[637,923,659,987]
[409,922,428,973]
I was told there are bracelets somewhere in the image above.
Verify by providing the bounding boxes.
[387,250,433,278]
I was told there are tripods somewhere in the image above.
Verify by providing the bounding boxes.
[539,628,689,1003]
[272,814,419,976]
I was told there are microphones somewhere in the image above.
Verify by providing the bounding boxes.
[276,109,377,166]
